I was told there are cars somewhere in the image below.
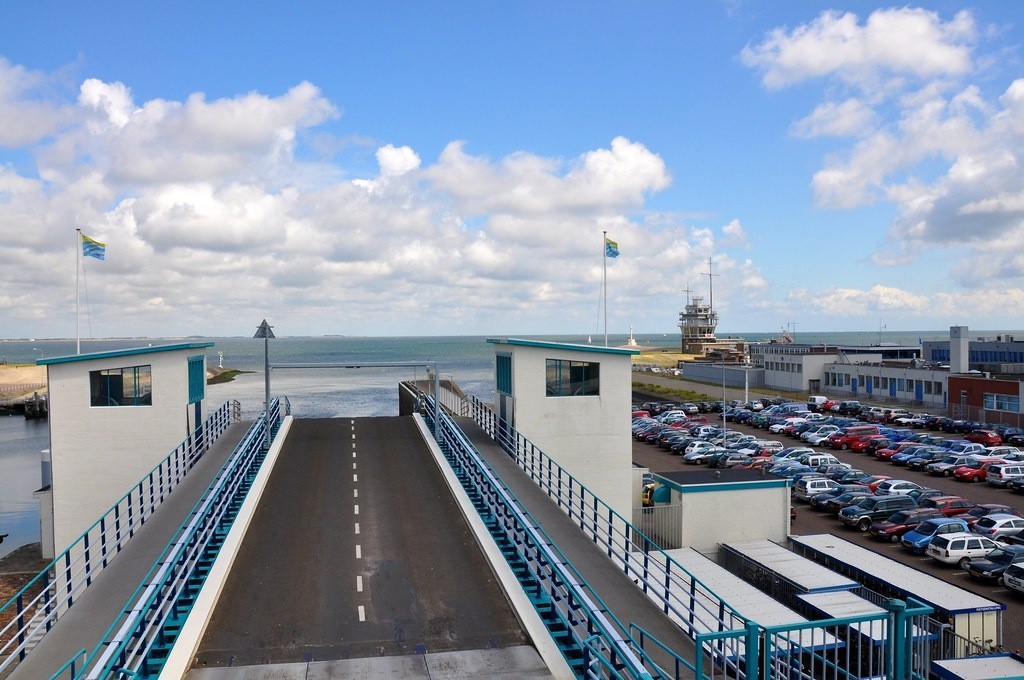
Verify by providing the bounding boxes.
[635,394,1024,596]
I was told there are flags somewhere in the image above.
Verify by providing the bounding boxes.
[605,237,620,258]
[81,234,106,261]
